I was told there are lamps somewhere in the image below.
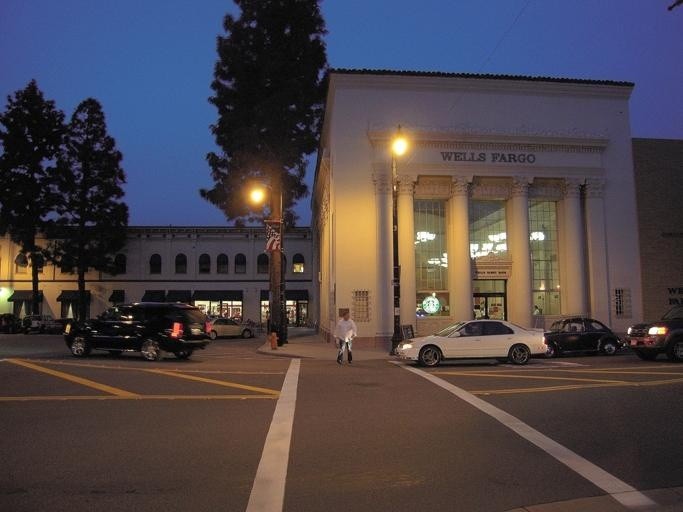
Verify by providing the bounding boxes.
[415,231,545,267]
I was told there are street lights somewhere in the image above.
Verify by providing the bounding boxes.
[246,181,289,344]
[391,125,413,355]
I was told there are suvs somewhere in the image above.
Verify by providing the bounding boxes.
[624,307,683,362]
[64,302,212,361]
[0,313,63,335]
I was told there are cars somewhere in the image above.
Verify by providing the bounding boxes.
[209,318,251,340]
[394,320,549,367]
[544,318,626,358]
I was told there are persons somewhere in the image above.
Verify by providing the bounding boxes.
[266,311,270,330]
[533,305,540,315]
[224,310,228,317]
[335,310,356,364]
[207,310,210,320]
